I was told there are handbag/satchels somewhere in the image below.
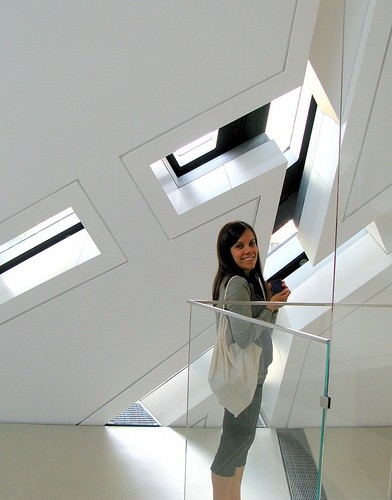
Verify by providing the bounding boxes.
[207,275,263,418]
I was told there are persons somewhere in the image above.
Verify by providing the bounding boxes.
[210,221,292,499]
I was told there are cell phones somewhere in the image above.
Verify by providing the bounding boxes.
[270,279,284,296]
